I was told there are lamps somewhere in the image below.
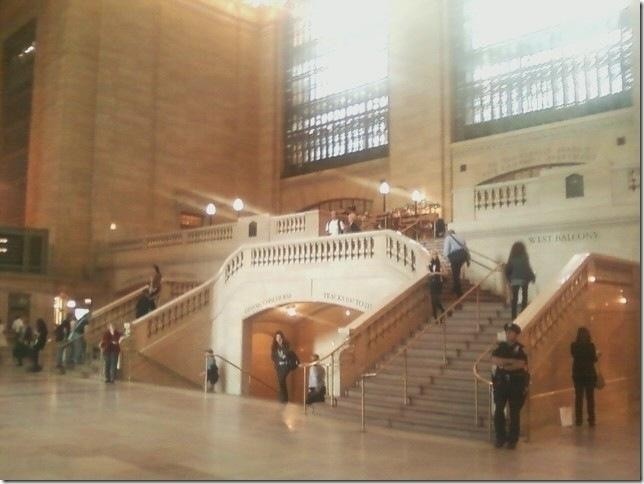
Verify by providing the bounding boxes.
[378,179,390,211]
[409,189,421,213]
[204,202,216,224]
[232,197,244,219]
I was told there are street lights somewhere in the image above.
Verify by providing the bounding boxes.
[379,176,390,217]
[66,298,78,319]
[231,196,244,219]
[412,189,421,243]
[205,200,218,227]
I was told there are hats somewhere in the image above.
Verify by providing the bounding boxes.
[503,322,520,333]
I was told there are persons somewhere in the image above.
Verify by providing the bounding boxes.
[147,262,159,306]
[324,209,342,236]
[486,321,529,448]
[427,251,452,325]
[0,312,86,376]
[443,228,470,309]
[569,326,603,426]
[198,348,218,393]
[306,352,326,405]
[135,287,155,319]
[344,205,358,232]
[97,321,123,383]
[270,330,294,401]
[503,240,536,319]
